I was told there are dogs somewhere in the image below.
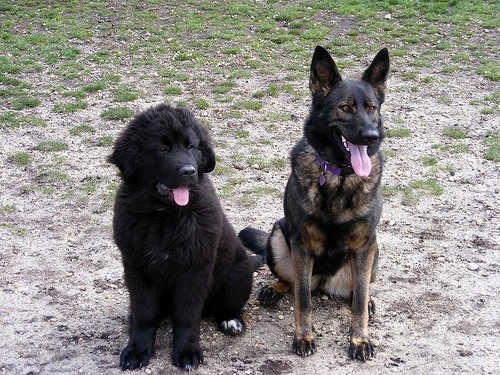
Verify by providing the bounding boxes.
[239,45,390,362]
[106,104,254,372]
